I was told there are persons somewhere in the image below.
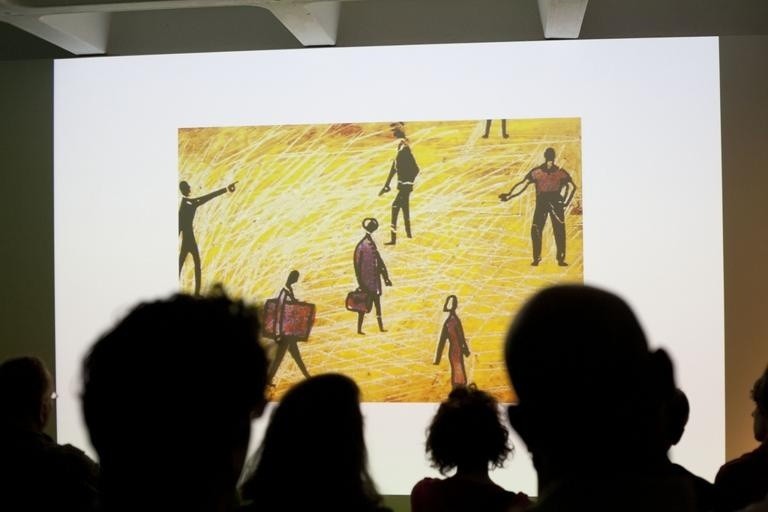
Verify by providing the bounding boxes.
[716,367,767,511]
[79,292,276,510]
[659,386,718,511]
[0,356,100,511]
[503,276,717,511]
[234,372,395,512]
[410,383,535,511]
